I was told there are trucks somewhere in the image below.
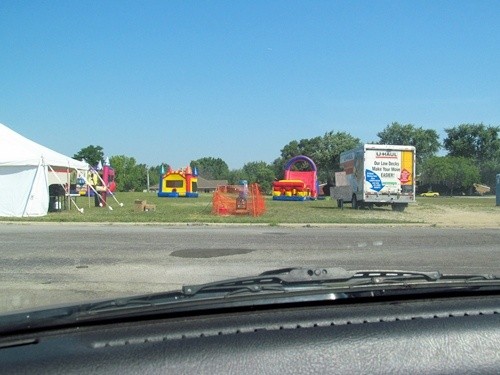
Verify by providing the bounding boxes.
[335,144,416,212]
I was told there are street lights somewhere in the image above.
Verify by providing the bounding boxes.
[145,164,149,191]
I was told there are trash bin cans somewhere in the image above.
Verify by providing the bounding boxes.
[94,190,107,208]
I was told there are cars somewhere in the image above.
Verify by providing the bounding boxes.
[420,190,439,197]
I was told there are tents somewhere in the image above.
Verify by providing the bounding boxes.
[0,121,91,218]
